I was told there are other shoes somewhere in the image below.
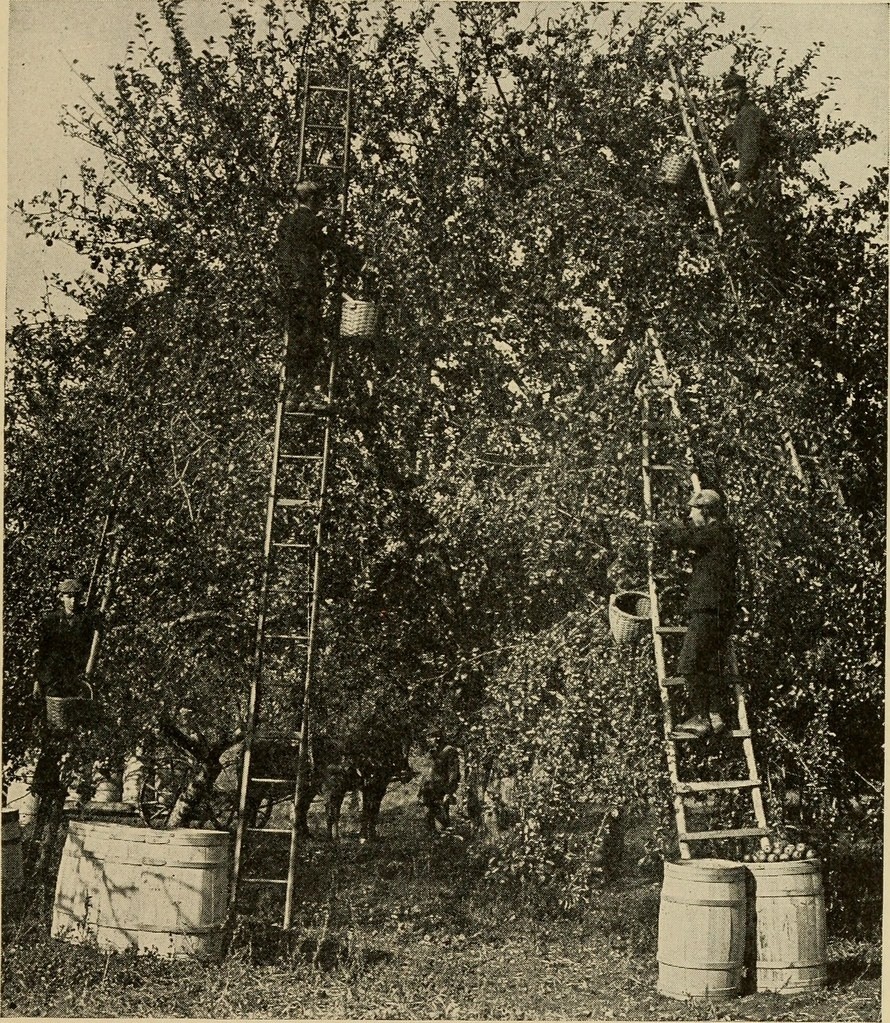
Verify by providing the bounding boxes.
[707,712,725,735]
[285,399,309,412]
[675,714,712,737]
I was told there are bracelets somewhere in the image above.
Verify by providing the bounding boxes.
[638,518,645,524]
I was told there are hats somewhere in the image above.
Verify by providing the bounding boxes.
[58,579,82,593]
[685,489,722,506]
[295,180,328,192]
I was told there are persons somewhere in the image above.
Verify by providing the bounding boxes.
[619,488,741,738]
[32,579,96,801]
[325,733,461,842]
[277,179,378,412]
[718,76,782,277]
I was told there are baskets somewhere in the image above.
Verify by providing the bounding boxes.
[609,589,662,643]
[340,291,379,339]
[44,677,93,730]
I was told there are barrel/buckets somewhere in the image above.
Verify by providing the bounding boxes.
[743,856,830,996]
[655,857,748,1002]
[0,807,24,896]
[49,820,230,968]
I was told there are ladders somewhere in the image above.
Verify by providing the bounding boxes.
[226,1,354,928]
[22,458,136,872]
[639,373,777,858]
